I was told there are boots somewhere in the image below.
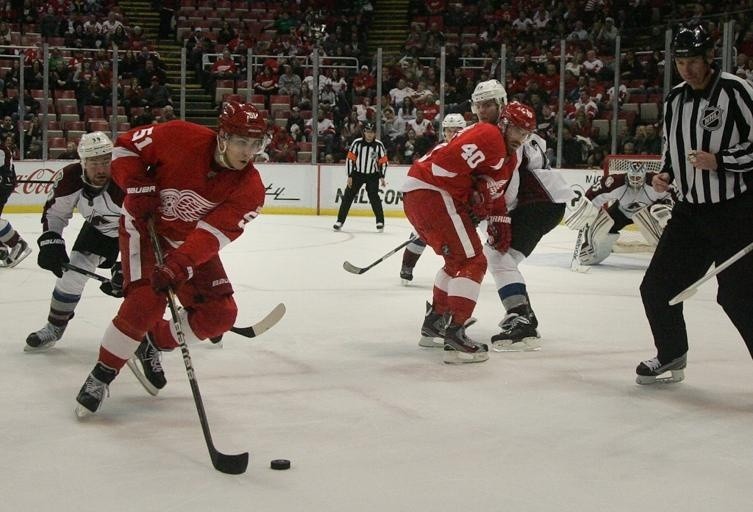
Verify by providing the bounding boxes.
[421,293,538,353]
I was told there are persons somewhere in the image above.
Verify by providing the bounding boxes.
[330,123,389,228]
[76,100,267,415]
[469,79,573,346]
[398,112,466,280]
[0,0,753,166]
[634,22,753,376]
[399,101,537,352]
[580,160,684,273]
[0,141,27,263]
[25,130,223,347]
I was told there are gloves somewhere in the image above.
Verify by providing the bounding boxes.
[100,262,124,298]
[38,231,70,278]
[488,218,512,254]
[150,252,198,296]
[124,176,161,232]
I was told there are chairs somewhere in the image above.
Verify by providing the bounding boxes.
[0,0,750,166]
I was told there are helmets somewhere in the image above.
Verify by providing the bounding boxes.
[77,131,113,168]
[0,124,14,149]
[670,19,713,57]
[442,79,535,138]
[217,100,267,155]
[627,160,646,187]
[362,120,377,133]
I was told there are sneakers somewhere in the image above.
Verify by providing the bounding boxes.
[26,311,75,347]
[0,235,27,264]
[400,262,413,280]
[334,222,342,229]
[76,360,117,412]
[134,332,166,389]
[376,222,383,229]
[636,353,686,376]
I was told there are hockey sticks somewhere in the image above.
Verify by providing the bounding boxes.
[60,262,286,338]
[343,235,415,275]
[145,211,249,475]
